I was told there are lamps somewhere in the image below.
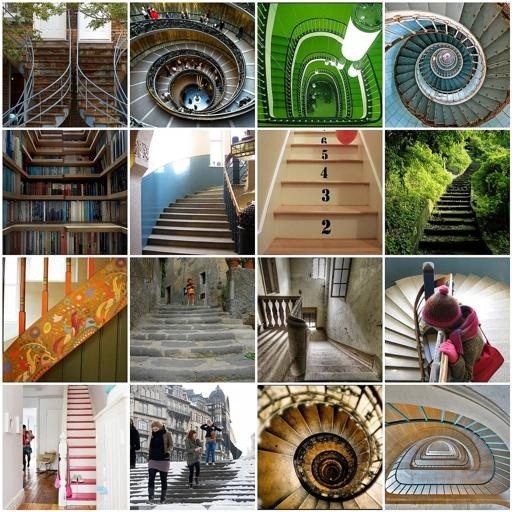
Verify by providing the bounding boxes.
[329,4,382,78]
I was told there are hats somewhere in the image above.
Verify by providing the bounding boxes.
[422,286,461,326]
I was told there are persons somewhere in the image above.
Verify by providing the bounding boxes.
[146,421,173,504]
[186,430,203,485]
[422,285,483,382]
[185,279,197,306]
[130,418,140,467]
[200,418,223,466]
[142,6,252,114]
[23,425,34,471]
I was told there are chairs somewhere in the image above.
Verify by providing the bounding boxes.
[38,451,57,476]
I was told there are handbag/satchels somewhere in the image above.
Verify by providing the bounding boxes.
[473,342,505,381]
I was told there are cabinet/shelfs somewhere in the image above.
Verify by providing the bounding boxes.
[2,130,126,255]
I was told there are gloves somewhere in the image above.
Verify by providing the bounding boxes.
[439,340,457,365]
